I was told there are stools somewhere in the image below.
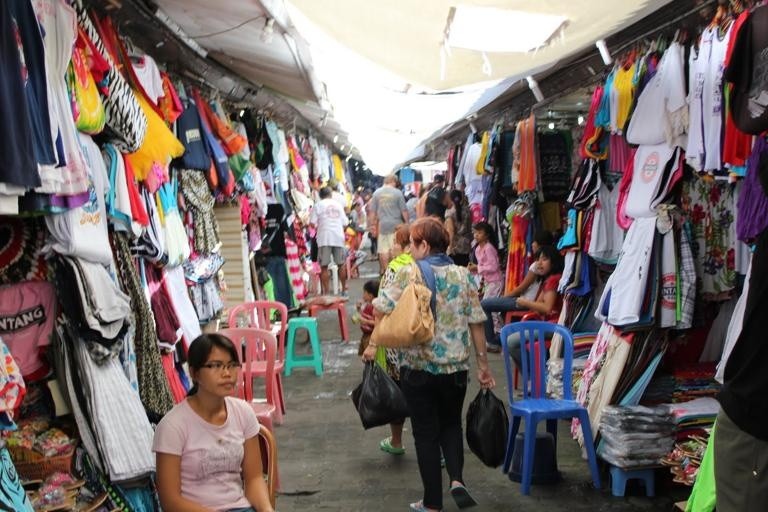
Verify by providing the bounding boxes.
[307,304,348,340]
[608,469,661,497]
[514,341,550,399]
[501,312,527,351]
[284,317,324,377]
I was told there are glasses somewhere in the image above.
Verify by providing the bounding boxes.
[201,360,242,370]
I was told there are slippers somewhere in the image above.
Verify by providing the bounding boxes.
[409,500,443,512]
[380,436,406,454]
[448,485,480,510]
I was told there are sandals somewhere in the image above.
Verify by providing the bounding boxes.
[440,457,446,468]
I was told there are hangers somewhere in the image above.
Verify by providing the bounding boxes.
[613,0,747,69]
[121,30,143,60]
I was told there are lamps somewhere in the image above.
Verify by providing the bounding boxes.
[257,19,277,43]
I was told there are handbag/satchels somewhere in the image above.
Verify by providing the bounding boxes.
[451,233,470,255]
[369,283,435,347]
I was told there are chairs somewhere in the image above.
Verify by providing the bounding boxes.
[227,301,288,424]
[499,320,603,493]
[215,328,278,432]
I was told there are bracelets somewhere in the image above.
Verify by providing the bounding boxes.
[476,352,487,356]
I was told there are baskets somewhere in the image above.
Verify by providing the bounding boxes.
[14,437,78,482]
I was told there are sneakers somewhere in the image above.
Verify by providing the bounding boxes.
[486,341,501,354]
[340,291,349,302]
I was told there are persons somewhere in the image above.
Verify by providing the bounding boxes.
[477,228,561,393]
[310,175,510,355]
[149,334,274,512]
[371,222,423,454]
[354,280,384,360]
[360,216,496,511]
